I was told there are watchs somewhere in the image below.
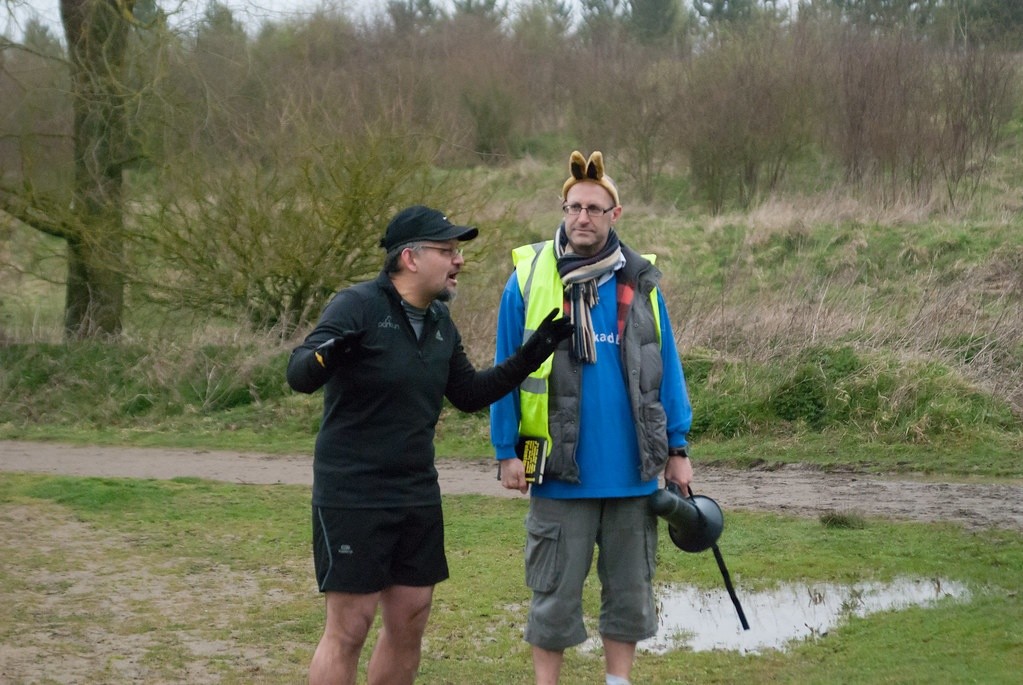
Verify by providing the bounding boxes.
[668,446,689,456]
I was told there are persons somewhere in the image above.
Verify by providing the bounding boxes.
[288,206,579,685]
[489,151,694,684]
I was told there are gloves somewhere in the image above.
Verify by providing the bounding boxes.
[315,330,385,368]
[521,306,575,373]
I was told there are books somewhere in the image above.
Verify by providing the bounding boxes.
[497,435,547,484]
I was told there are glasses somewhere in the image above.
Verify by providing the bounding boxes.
[408,244,465,258]
[561,202,619,216]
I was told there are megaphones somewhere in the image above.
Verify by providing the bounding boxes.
[649,481,724,553]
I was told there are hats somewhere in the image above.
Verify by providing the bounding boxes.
[375,205,479,249]
